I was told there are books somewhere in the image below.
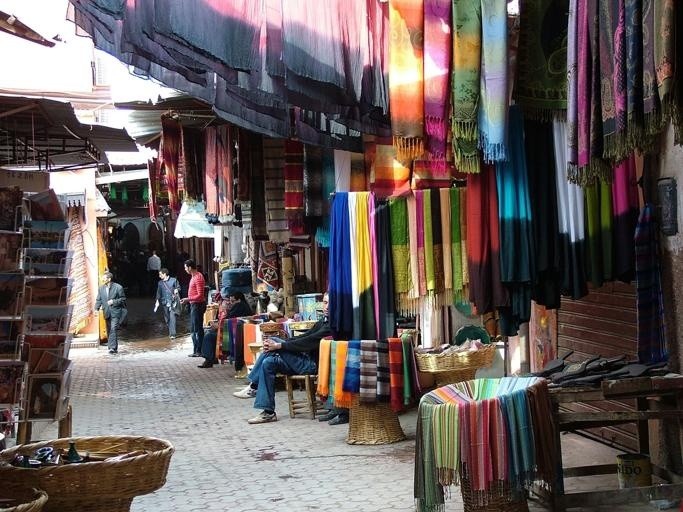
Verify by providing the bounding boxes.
[0,180,69,420]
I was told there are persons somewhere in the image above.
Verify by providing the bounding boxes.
[154,268,182,336]
[180,259,205,357]
[117,250,160,299]
[96,274,126,355]
[197,291,252,368]
[319,411,347,425]
[232,292,335,424]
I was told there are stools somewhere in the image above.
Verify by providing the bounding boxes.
[284,372,331,420]
[221,358,233,365]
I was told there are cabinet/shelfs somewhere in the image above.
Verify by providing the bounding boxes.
[0,195,74,425]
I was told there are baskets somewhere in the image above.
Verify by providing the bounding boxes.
[402,328,417,344]
[455,459,531,511]
[0,481,50,511]
[415,343,496,386]
[343,391,407,445]
[0,436,175,512]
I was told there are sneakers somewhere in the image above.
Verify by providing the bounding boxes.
[232,384,257,399]
[170,335,176,339]
[248,408,277,424]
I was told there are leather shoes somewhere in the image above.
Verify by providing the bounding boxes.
[328,415,349,425]
[188,353,219,368]
[109,348,115,353]
[319,410,339,421]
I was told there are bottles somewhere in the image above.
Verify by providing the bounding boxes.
[67,442,79,461]
[258,291,270,313]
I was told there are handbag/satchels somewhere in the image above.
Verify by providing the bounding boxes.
[172,298,183,316]
[118,298,128,329]
